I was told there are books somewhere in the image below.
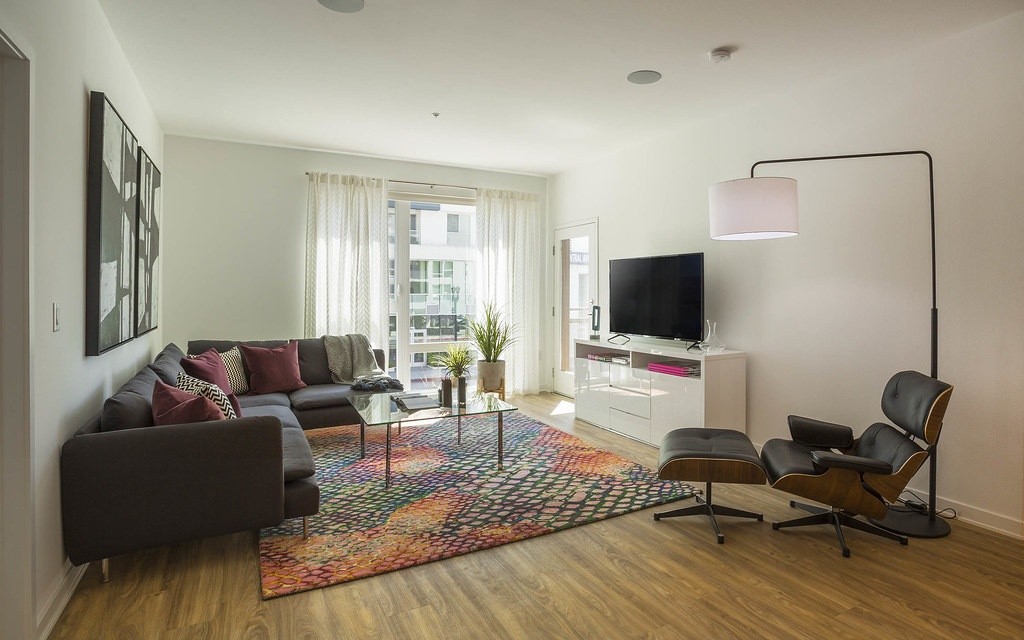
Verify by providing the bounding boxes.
[390,393,439,410]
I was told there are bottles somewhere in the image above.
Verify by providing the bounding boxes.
[698,319,726,354]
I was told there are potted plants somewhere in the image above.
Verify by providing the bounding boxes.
[461,297,519,391]
[433,342,476,387]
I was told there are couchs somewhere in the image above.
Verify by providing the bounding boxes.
[59,337,386,584]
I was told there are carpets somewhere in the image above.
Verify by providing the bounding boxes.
[257,409,703,600]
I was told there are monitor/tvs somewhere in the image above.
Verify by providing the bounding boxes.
[606,251,705,352]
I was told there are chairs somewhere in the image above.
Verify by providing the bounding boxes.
[762,370,954,558]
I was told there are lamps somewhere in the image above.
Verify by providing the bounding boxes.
[708,150,951,539]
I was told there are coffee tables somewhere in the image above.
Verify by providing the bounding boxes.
[345,389,518,490]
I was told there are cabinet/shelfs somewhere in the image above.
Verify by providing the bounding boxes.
[574,338,746,449]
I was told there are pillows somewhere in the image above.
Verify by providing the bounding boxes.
[240,341,307,396]
[187,346,249,396]
[180,348,232,395]
[151,380,225,425]
[175,372,237,420]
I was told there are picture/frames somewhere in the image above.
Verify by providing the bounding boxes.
[85,90,161,356]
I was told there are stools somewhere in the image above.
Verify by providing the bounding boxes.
[654,428,766,544]
